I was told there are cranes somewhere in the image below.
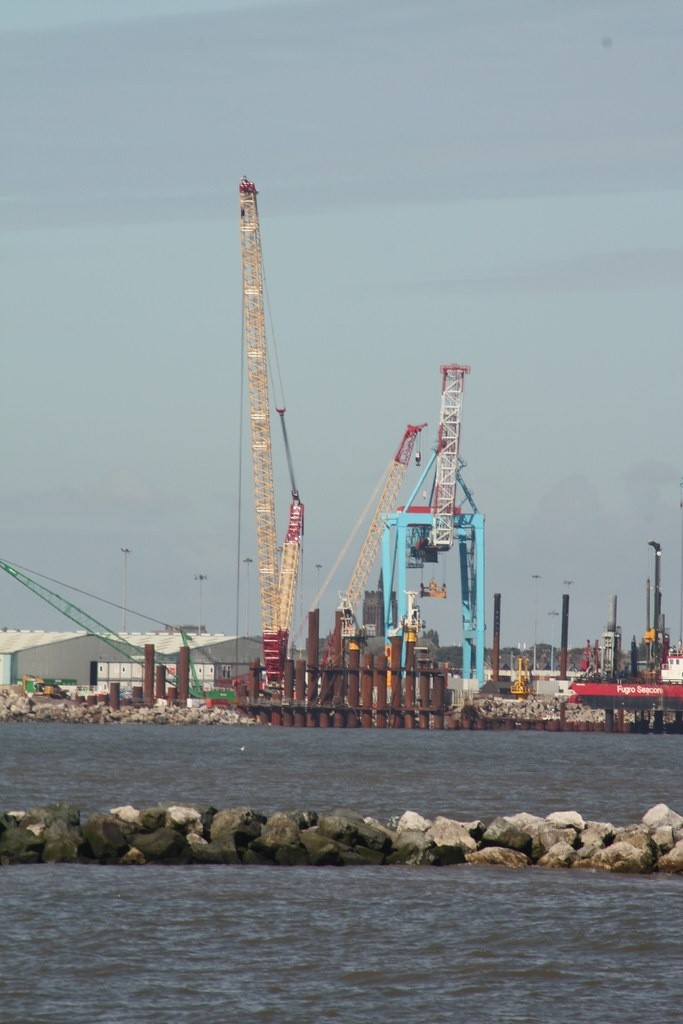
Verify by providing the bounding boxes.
[1,176,683,731]
